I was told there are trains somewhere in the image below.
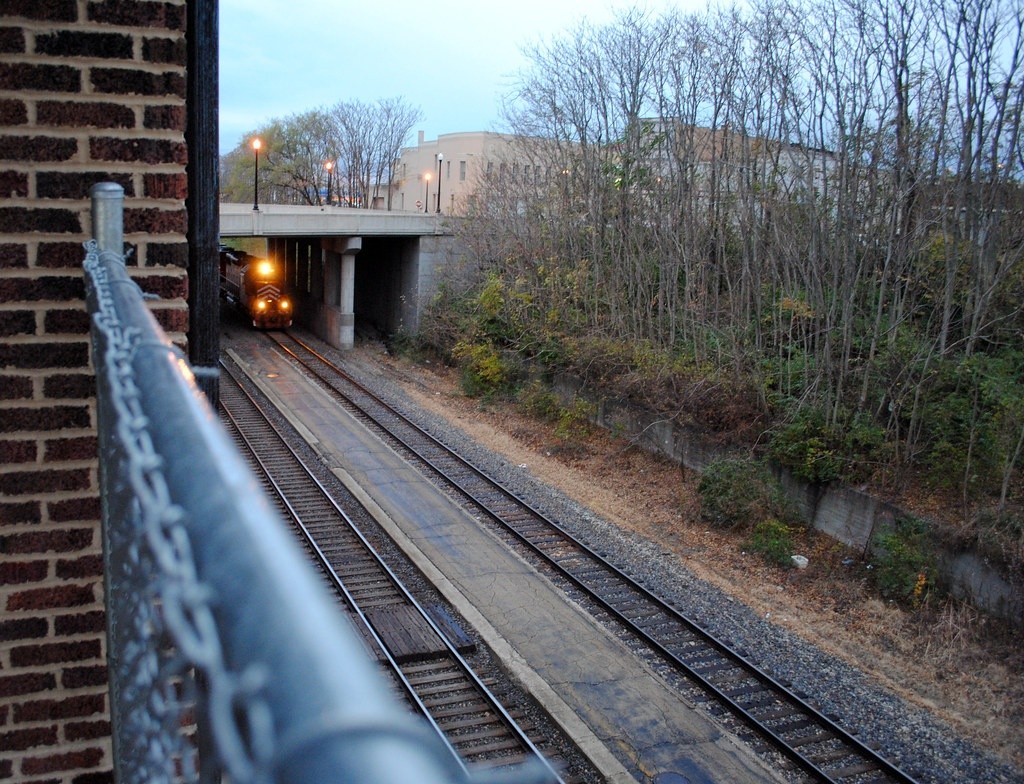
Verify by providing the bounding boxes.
[219,242,296,329]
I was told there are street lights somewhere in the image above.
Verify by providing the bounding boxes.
[253,139,261,211]
[436,153,444,213]
[325,161,333,205]
[425,173,432,213]
